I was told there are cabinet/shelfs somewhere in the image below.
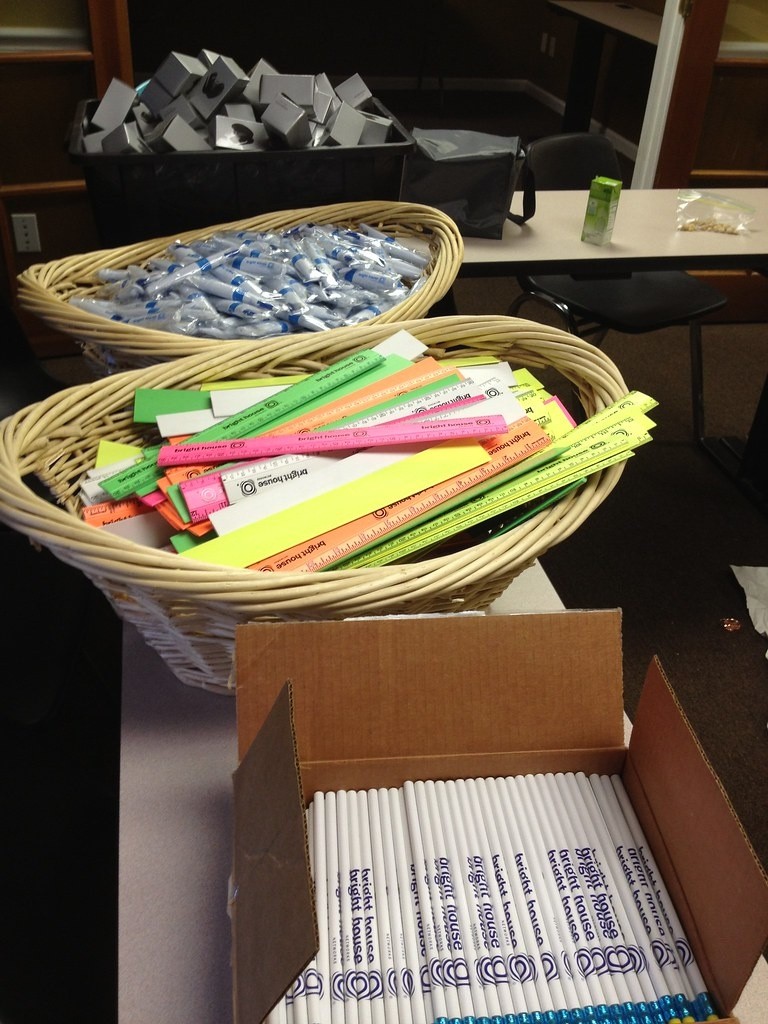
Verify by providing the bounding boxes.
[0,0,135,361]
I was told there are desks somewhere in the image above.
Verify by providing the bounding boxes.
[549,1,768,153]
[417,187,768,317]
[120,552,768,1024]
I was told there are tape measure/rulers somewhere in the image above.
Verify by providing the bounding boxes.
[77,347,658,573]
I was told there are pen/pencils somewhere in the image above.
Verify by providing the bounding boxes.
[71,219,429,338]
[267,772,718,1023]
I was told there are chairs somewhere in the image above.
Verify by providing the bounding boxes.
[505,134,727,449]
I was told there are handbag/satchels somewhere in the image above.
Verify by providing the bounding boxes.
[399,128,537,240]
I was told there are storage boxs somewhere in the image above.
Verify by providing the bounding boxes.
[225,608,768,1024]
[68,83,417,246]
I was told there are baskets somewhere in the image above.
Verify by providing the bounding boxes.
[0,315,633,695]
[16,201,466,376]
